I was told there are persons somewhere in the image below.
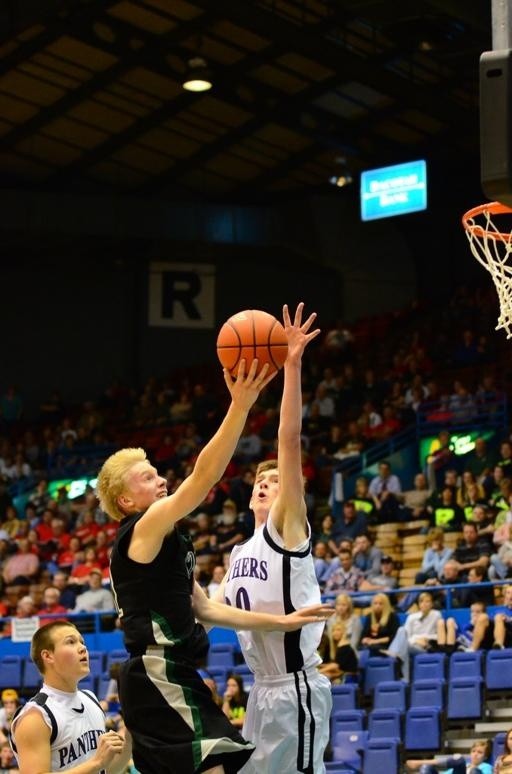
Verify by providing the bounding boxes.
[0,302,512,774]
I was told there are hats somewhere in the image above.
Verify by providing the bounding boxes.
[379,555,393,562]
[89,567,103,577]
[1,689,19,703]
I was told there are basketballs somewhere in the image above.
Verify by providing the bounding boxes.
[217,310,288,380]
